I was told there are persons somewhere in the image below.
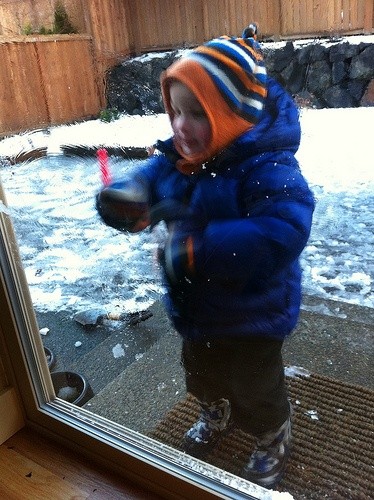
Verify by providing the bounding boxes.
[95,21,314,488]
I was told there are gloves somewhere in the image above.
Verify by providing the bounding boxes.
[97,188,148,233]
[163,235,200,287]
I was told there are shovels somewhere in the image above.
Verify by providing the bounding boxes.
[74,308,153,327]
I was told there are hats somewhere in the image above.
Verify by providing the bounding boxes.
[160,22,269,163]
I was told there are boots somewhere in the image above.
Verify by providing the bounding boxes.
[184,398,232,451]
[244,401,292,482]
